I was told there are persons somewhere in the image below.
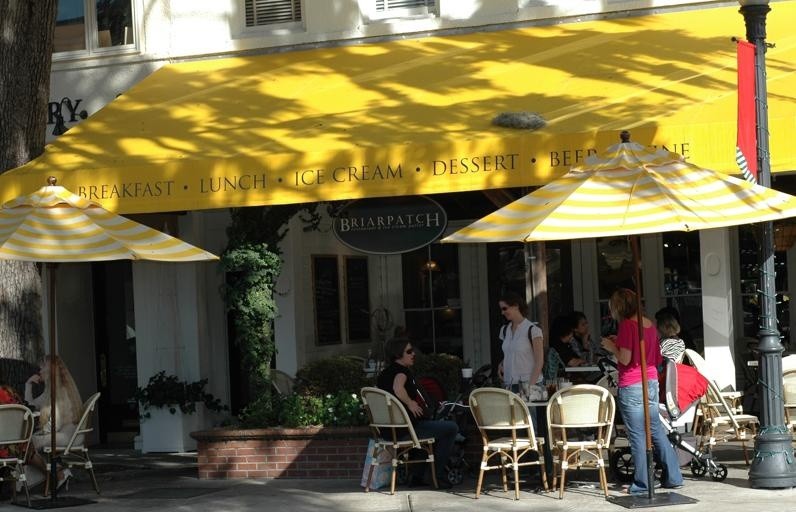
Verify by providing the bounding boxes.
[498,289,553,488]
[543,346,565,378]
[600,288,684,493]
[557,307,686,381]
[22,355,87,492]
[373,335,458,489]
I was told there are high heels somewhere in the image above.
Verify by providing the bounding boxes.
[55,468,74,489]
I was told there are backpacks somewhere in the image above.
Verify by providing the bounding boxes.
[503,323,566,379]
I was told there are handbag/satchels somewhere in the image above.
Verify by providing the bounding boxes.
[361,438,408,490]
[406,376,446,419]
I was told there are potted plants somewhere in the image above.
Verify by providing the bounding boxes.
[131,370,231,454]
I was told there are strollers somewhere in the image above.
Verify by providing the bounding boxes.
[411,363,496,484]
[597,358,728,482]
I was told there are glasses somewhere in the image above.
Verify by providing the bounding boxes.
[502,306,510,311]
[404,348,412,354]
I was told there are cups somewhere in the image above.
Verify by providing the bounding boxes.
[461,368,473,378]
[518,377,569,401]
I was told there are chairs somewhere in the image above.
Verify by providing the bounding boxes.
[449,362,495,417]
[362,386,441,492]
[543,384,617,494]
[32,392,102,495]
[686,350,744,435]
[271,369,295,396]
[695,375,761,463]
[0,403,34,506]
[469,385,549,495]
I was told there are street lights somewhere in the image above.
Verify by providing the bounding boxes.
[738,0,795,490]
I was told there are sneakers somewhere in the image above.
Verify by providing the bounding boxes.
[437,478,453,489]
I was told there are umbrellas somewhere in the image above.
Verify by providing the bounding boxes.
[434,130,796,500]
[0,175,220,498]
